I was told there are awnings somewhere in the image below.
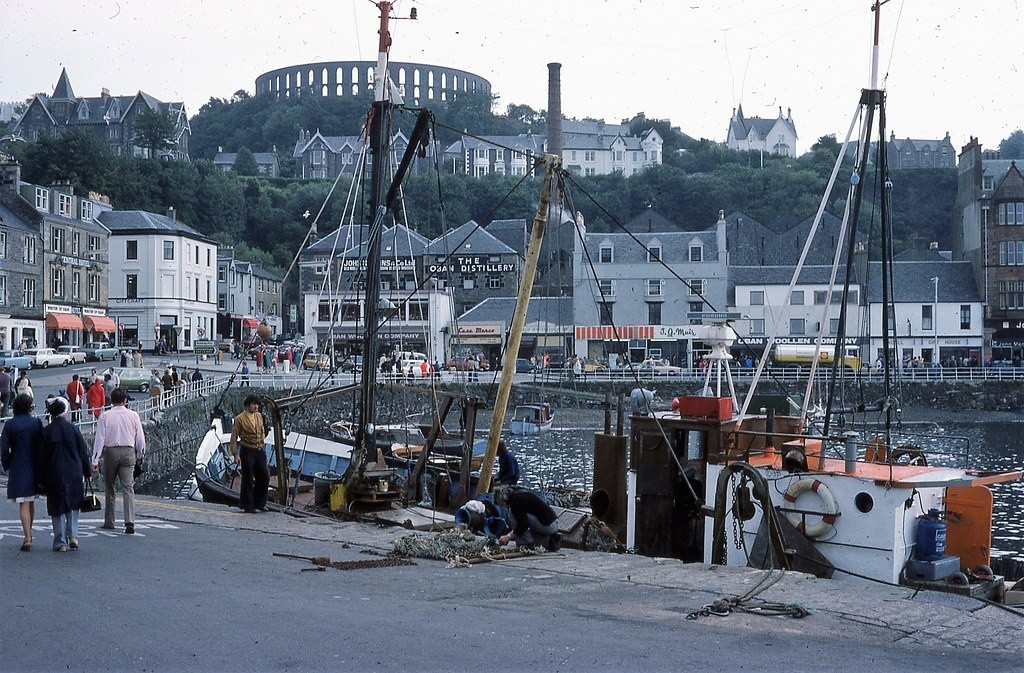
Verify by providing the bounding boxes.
[46,313,83,331]
[243,319,258,329]
[84,316,116,334]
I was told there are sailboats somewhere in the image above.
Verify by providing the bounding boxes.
[187,1,561,530]
[478,0,1022,603]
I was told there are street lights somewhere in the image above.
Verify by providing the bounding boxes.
[931,276,940,365]
[439,325,450,371]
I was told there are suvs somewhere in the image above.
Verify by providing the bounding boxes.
[79,367,152,392]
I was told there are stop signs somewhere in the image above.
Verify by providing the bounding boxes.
[154,325,160,333]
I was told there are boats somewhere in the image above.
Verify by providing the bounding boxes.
[329,419,488,458]
[390,442,486,472]
[510,402,555,436]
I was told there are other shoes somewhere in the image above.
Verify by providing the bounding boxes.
[518,543,538,551]
[548,533,563,552]
[125,523,134,533]
[257,506,268,512]
[100,524,114,529]
[244,506,260,513]
[257,369,278,374]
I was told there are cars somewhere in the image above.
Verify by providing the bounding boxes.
[583,358,606,370]
[516,359,539,374]
[240,333,308,367]
[341,355,363,373]
[21,348,70,369]
[0,349,35,370]
[83,342,120,362]
[302,353,336,371]
[448,356,475,371]
[623,359,681,376]
[56,345,87,365]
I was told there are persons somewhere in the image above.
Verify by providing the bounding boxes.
[1,394,45,551]
[48,336,69,351]
[17,337,38,355]
[1,365,36,416]
[530,351,763,376]
[378,352,480,385]
[231,394,271,513]
[86,378,106,429]
[491,484,563,552]
[87,367,134,409]
[248,330,342,376]
[238,362,250,388]
[118,349,144,369]
[230,342,245,359]
[872,353,1024,373]
[131,334,143,353]
[153,334,170,357]
[67,374,85,424]
[493,440,521,485]
[148,364,204,409]
[93,388,147,533]
[35,398,92,554]
[95,334,115,348]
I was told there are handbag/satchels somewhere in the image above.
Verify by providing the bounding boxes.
[81,479,101,513]
[75,394,83,404]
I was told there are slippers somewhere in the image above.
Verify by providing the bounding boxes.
[58,545,67,551]
[68,542,78,550]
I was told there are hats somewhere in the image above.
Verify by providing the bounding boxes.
[382,353,385,356]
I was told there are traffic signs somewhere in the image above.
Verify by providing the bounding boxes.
[193,340,218,356]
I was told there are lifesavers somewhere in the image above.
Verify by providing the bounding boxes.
[891,446,926,466]
[784,478,836,541]
[865,440,885,463]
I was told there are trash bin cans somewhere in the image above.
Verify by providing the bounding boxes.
[202,354,207,361]
[219,351,223,361]
[314,471,342,507]
[283,360,290,374]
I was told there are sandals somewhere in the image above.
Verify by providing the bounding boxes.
[20,540,30,551]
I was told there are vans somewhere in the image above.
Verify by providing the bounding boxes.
[398,352,429,368]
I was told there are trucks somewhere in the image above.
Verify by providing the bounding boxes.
[773,343,862,372]
